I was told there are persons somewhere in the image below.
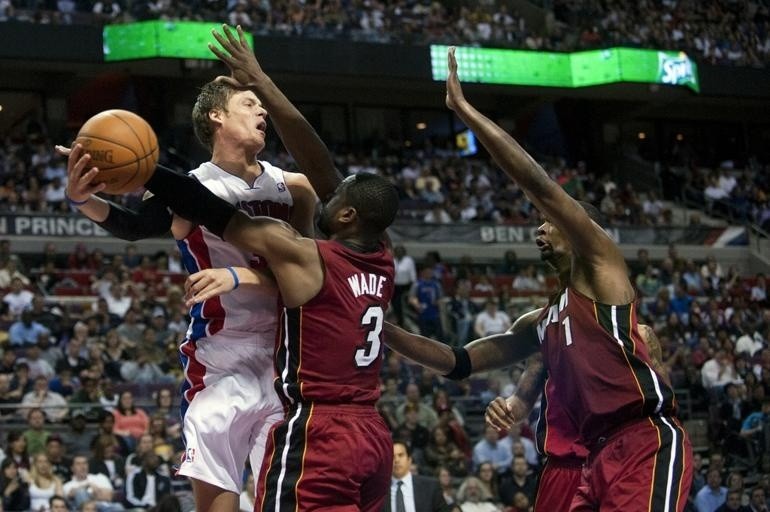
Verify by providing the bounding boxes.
[1,0,770,511]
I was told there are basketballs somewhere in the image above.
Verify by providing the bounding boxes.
[75,110,160,197]
